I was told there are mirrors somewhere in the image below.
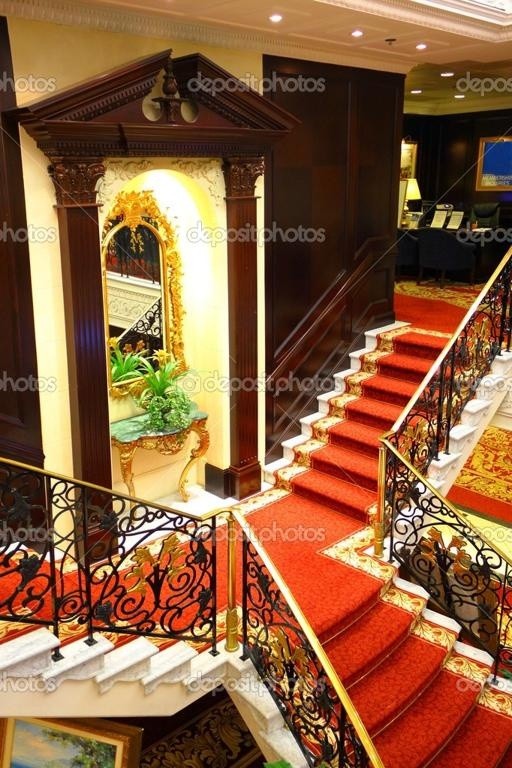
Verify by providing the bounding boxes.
[99,189,186,402]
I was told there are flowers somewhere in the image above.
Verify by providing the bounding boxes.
[107,336,153,382]
[114,349,192,432]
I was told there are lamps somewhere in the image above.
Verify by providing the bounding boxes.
[404,178,423,212]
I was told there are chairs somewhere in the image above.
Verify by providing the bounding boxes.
[468,201,502,227]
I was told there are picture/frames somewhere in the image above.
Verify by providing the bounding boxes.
[0,718,145,768]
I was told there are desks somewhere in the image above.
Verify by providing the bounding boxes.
[111,401,211,524]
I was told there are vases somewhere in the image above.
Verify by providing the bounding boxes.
[160,406,174,423]
